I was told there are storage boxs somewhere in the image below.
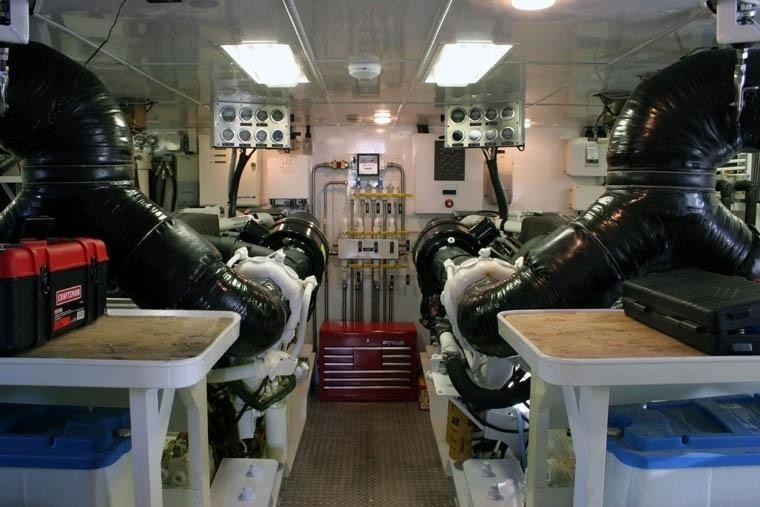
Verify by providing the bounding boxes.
[600,391,760,506]
[0,403,137,506]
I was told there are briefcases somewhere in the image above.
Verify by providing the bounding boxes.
[621,269,760,355]
[0,235,110,356]
